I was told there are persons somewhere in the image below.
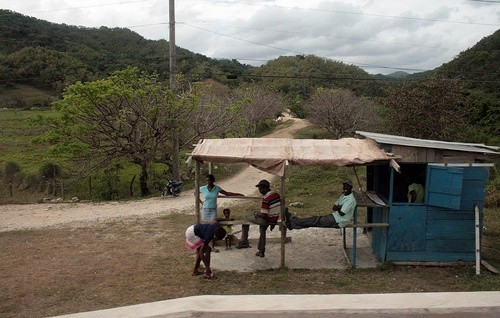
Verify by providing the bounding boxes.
[406,170,424,203]
[284,179,357,231]
[213,208,234,249]
[185,223,227,279]
[193,174,245,252]
[235,179,281,258]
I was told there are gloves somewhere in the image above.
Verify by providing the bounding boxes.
[270,224,275,231]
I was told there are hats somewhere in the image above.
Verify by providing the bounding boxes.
[342,180,353,186]
[255,179,270,187]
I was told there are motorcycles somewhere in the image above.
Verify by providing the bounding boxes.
[160,178,184,200]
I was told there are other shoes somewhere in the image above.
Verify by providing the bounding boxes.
[255,251,265,258]
[285,207,291,221]
[203,272,218,280]
[285,220,293,231]
[192,271,202,276]
[211,247,219,253]
[235,242,249,249]
